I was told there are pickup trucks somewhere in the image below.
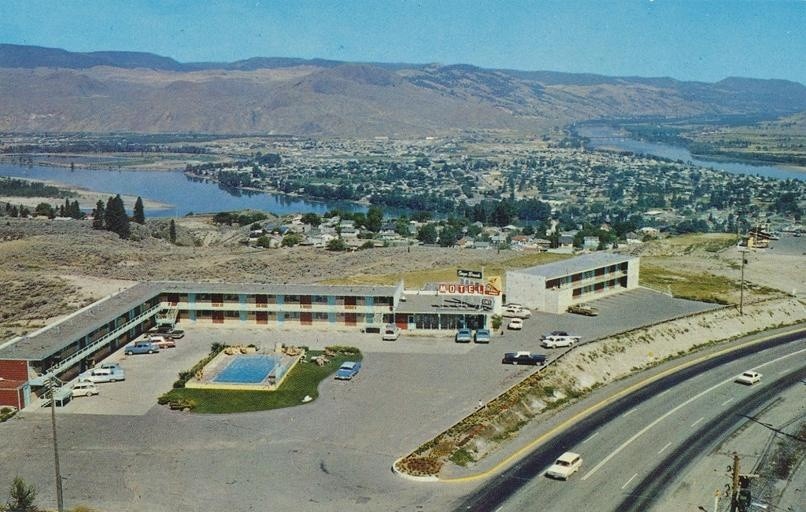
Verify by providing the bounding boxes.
[567,302,599,316]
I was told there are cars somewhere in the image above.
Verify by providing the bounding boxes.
[334,361,361,380]
[71,363,126,398]
[539,330,582,349]
[502,302,531,330]
[383,326,400,341]
[124,326,185,356]
[545,452,583,481]
[735,371,763,385]
[502,351,549,367]
[456,329,490,343]
